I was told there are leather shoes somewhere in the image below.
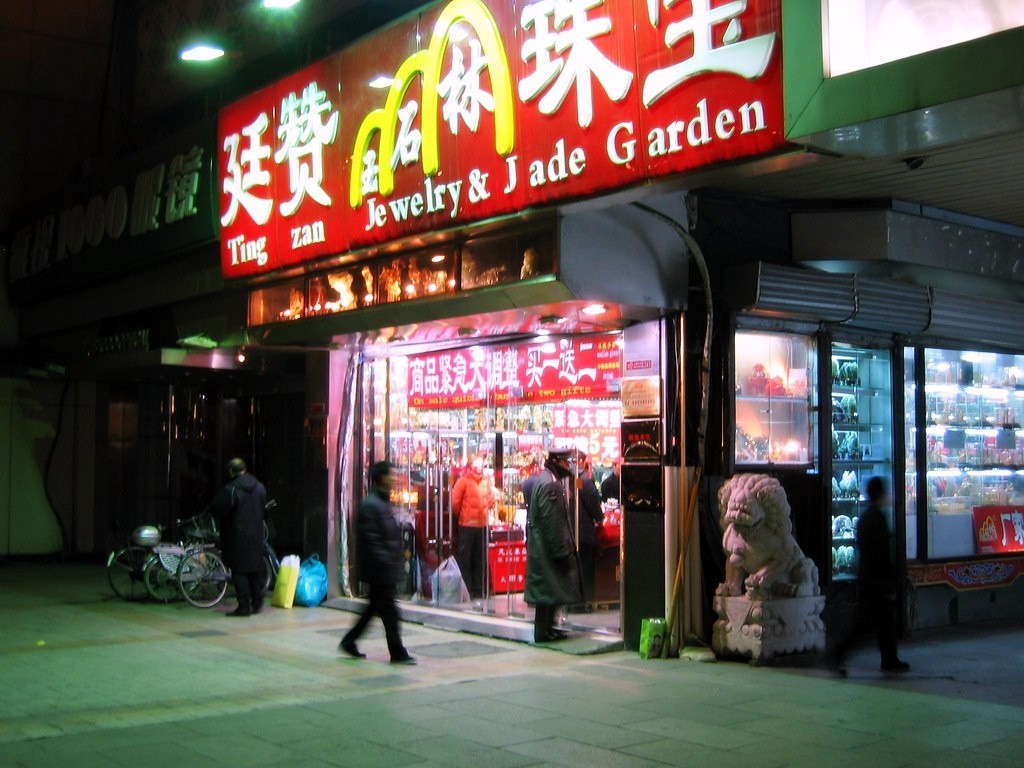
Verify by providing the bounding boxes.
[535,628,568,643]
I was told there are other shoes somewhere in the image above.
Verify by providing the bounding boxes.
[227,602,263,617]
[880,660,911,673]
[339,638,367,659]
[390,649,416,665]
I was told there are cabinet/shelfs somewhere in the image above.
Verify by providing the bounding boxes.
[818,381,891,581]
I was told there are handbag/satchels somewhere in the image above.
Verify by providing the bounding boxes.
[639,618,666,660]
[431,555,473,610]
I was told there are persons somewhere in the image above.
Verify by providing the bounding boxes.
[211,458,269,616]
[817,474,914,676]
[338,461,417,666]
[523,446,605,643]
[452,454,495,599]
[601,458,620,505]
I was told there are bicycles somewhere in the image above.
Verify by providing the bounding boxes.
[106,514,220,605]
[177,499,280,609]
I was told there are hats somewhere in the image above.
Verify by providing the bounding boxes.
[548,449,573,465]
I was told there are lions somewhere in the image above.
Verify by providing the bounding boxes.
[715,472,821,599]
